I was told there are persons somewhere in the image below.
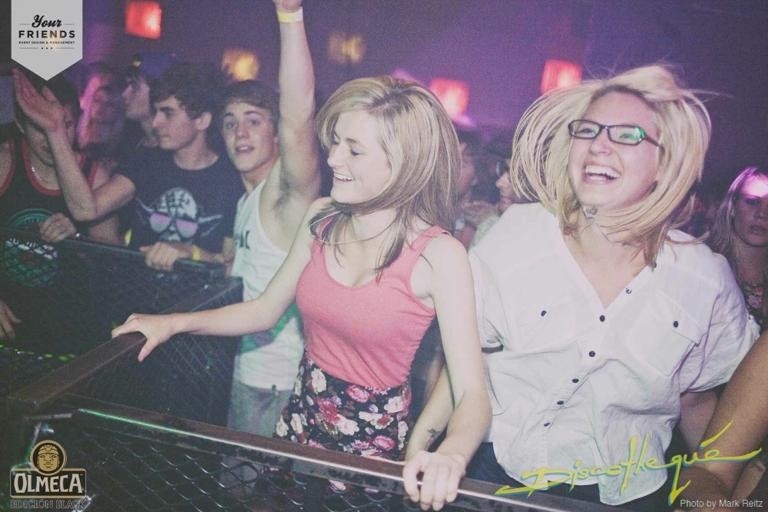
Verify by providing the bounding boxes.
[2,2,766,508]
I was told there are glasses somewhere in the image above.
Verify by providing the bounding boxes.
[568,120,662,149]
